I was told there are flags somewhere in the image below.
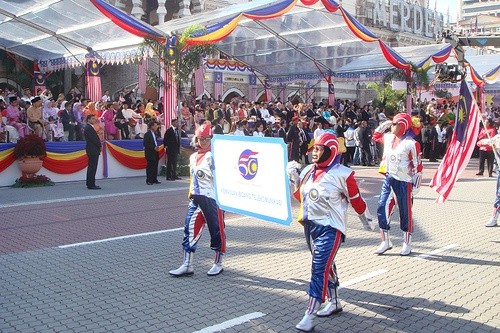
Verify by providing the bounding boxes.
[429,80,480,202]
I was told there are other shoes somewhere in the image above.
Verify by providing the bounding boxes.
[135,135,143,139]
[476,172,483,175]
[154,181,161,184]
[146,180,152,185]
[86,184,101,189]
[122,136,131,140]
[166,177,175,181]
[174,177,181,179]
[489,175,492,177]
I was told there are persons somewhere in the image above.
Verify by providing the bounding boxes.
[163,119,181,181]
[287,131,367,332]
[485,135,500,227]
[84,114,102,190]
[409,97,500,162]
[0,87,165,143]
[477,119,497,176]
[168,123,227,275]
[144,121,161,185]
[177,93,396,166]
[372,113,423,255]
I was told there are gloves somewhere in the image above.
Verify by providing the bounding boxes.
[412,186,418,196]
[383,119,393,128]
[287,160,302,183]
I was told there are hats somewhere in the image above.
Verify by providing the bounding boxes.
[393,112,412,134]
[9,96,17,100]
[313,132,339,167]
[194,126,213,139]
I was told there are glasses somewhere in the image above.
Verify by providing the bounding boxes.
[199,137,210,141]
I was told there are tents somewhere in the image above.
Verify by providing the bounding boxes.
[0,0,171,98]
[416,54,500,97]
[153,0,413,96]
[334,43,487,110]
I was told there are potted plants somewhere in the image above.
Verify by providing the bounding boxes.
[14,134,47,178]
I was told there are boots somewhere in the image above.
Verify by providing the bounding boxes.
[316,287,343,317]
[484,206,499,227]
[295,297,321,332]
[400,231,412,256]
[373,228,392,254]
[207,251,224,276]
[168,250,194,276]
